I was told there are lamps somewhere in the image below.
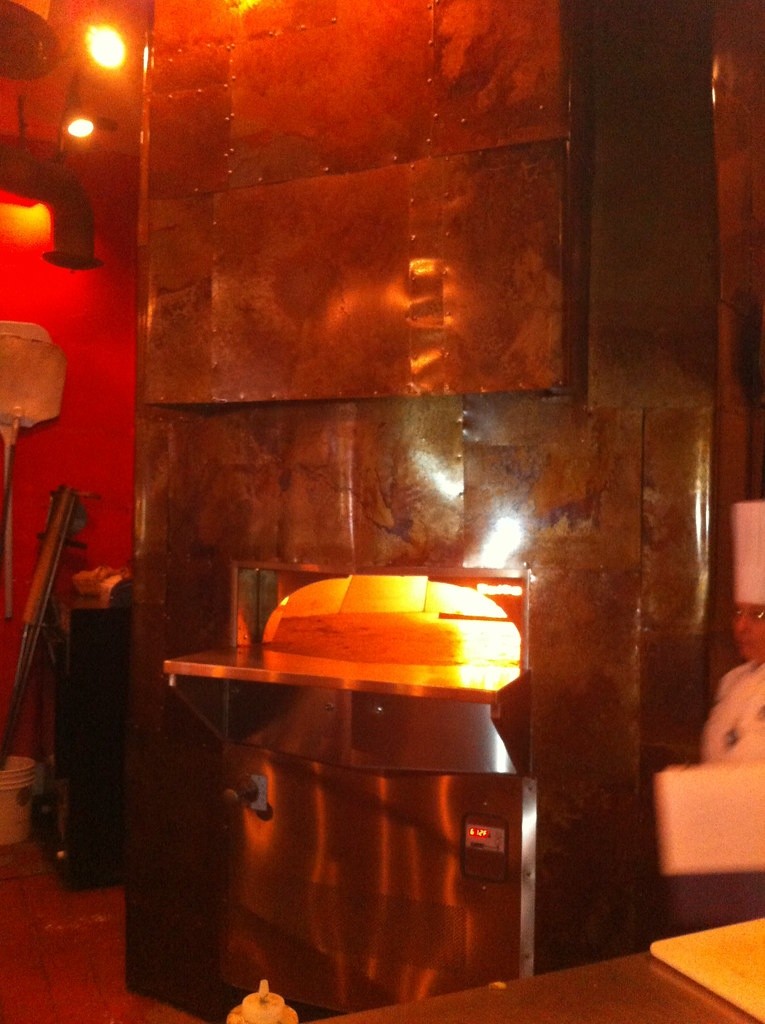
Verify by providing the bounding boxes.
[64,87,95,140]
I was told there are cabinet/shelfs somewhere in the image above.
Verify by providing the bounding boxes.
[30,584,135,891]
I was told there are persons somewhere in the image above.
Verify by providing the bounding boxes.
[701,499,765,762]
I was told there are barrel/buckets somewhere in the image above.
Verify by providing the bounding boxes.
[0,756,36,847]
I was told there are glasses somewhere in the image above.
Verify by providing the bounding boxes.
[733,609,765,622]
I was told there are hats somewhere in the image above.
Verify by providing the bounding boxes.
[732,499,765,604]
